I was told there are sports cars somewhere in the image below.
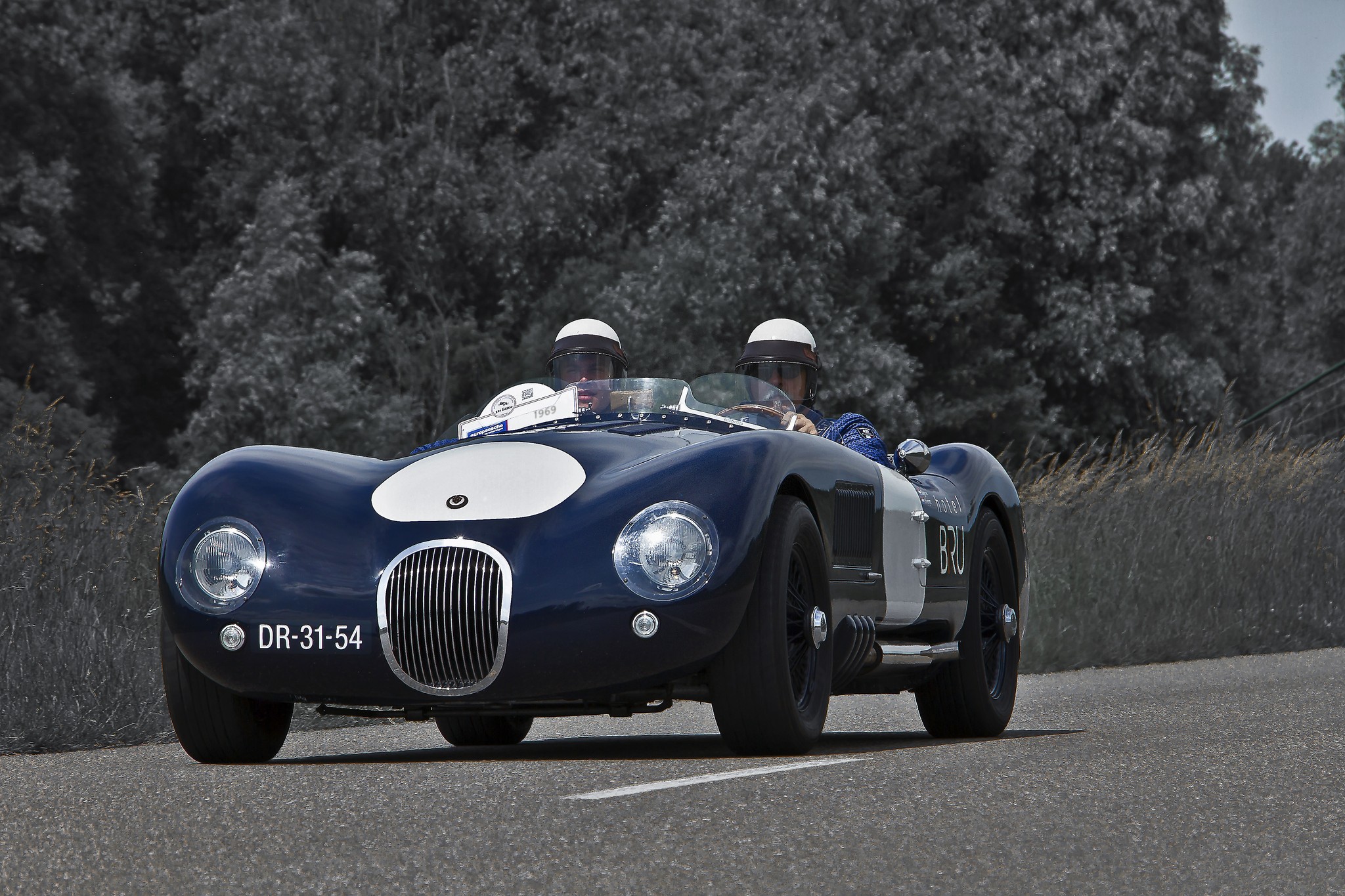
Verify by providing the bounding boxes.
[155,370,1029,765]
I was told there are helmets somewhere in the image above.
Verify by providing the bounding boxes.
[545,318,629,388]
[735,318,822,407]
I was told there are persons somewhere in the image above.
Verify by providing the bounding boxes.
[546,319,628,421]
[735,319,893,470]
